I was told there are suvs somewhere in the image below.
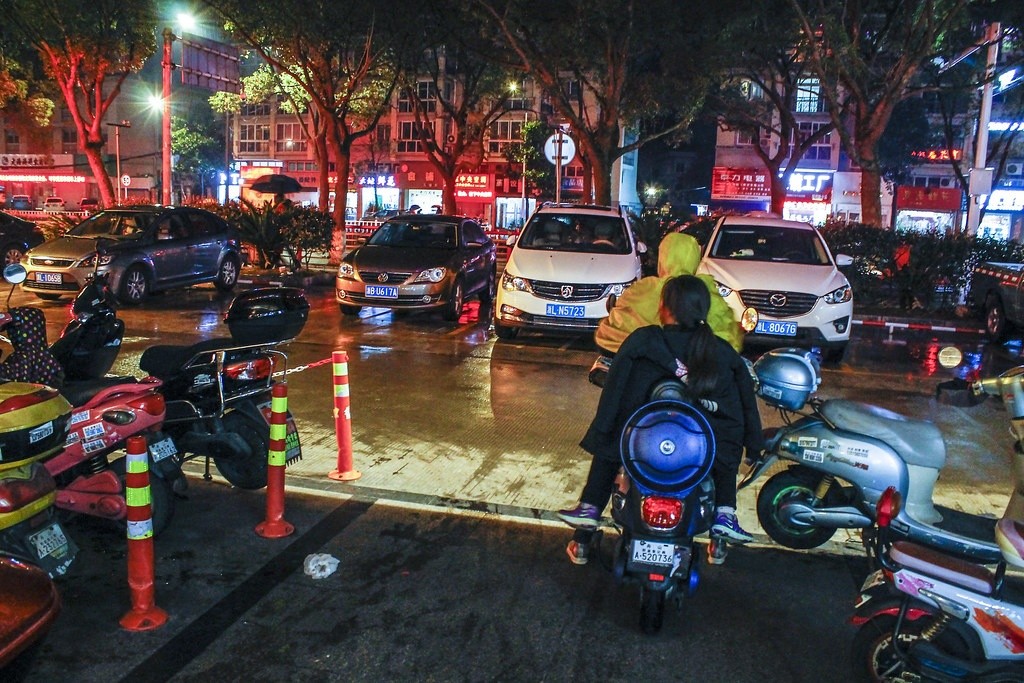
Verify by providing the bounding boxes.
[493,201,648,340]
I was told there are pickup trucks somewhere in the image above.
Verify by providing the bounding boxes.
[971,262,1024,342]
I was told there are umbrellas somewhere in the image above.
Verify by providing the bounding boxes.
[250,173,302,194]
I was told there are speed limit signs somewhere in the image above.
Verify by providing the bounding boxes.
[121,173,131,187]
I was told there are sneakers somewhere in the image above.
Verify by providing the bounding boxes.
[712,511,755,544]
[557,504,601,528]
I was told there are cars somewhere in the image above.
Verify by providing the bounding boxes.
[693,214,854,365]
[335,214,497,322]
[0,211,44,278]
[360,210,407,221]
[77,198,99,211]
[10,195,35,211]
[18,204,245,309]
[42,197,67,211]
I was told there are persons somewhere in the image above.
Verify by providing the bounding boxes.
[558,231,754,548]
[565,274,764,567]
[272,193,283,213]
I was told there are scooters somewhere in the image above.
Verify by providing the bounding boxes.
[736,345,1024,565]
[0,336,80,580]
[48,240,311,489]
[849,486,1024,683]
[605,292,760,636]
[0,263,191,540]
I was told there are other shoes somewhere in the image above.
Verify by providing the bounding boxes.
[708,540,729,563]
[565,539,590,565]
